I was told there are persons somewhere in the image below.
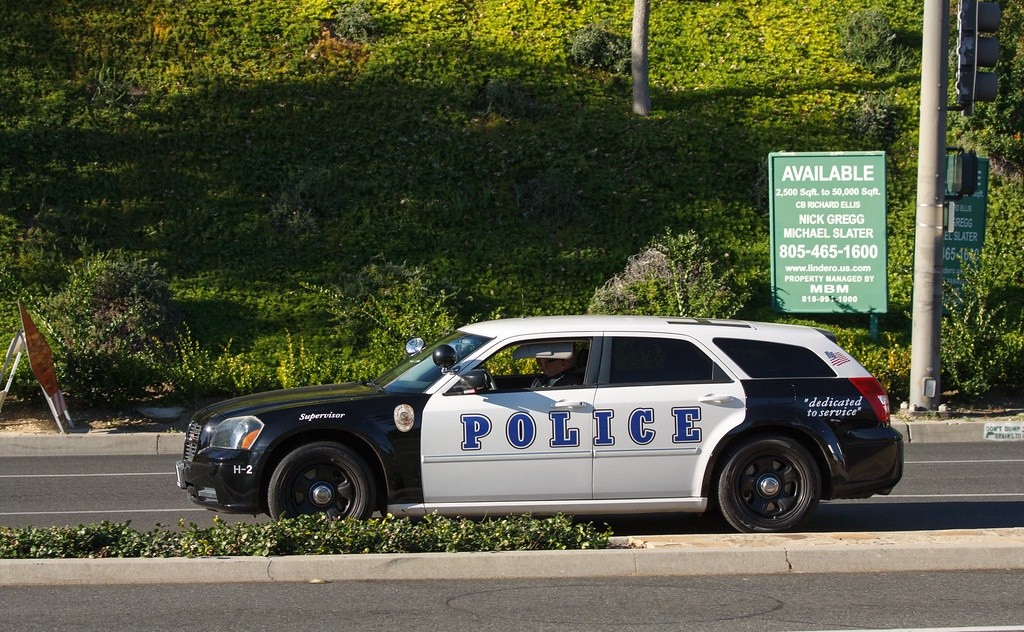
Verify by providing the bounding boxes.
[526,346,584,389]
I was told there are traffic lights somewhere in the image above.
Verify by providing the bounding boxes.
[973,1,1001,110]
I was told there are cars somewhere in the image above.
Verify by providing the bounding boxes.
[175,316,904,532]
